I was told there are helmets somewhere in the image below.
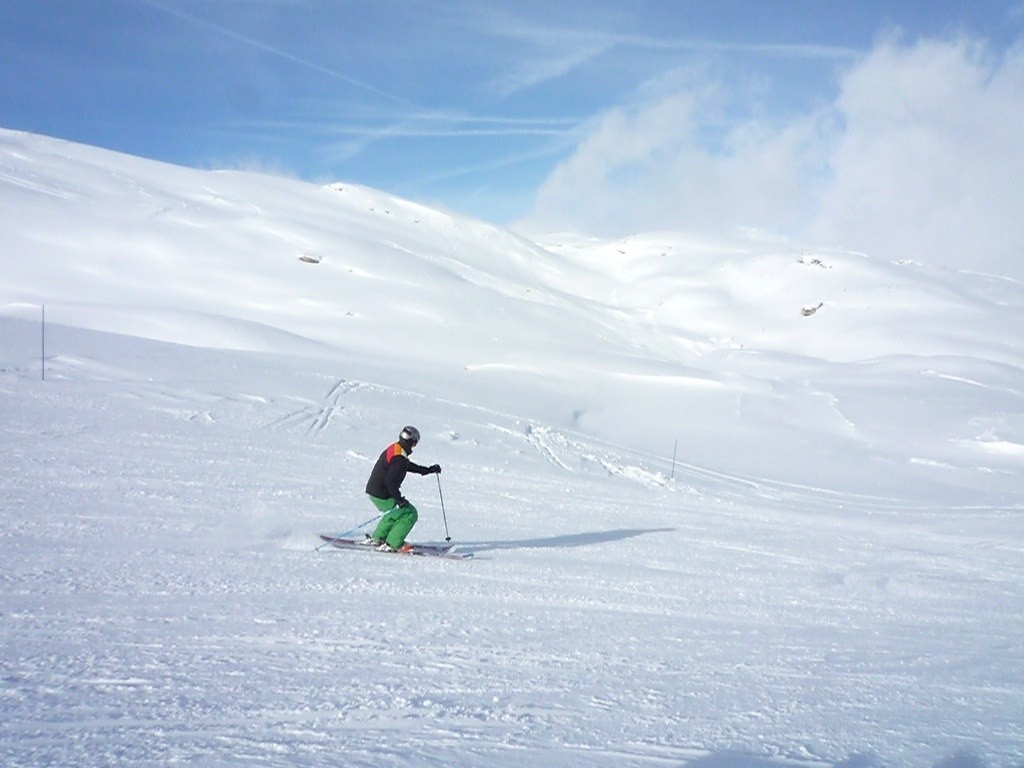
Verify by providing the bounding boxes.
[399,426,421,455]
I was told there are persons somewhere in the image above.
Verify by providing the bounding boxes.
[365,425,442,552]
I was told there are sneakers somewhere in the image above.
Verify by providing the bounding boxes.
[386,543,415,551]
[370,537,386,546]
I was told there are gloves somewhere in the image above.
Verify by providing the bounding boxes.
[397,497,408,508]
[428,464,441,473]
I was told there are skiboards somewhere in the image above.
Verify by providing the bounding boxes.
[319,534,475,561]
